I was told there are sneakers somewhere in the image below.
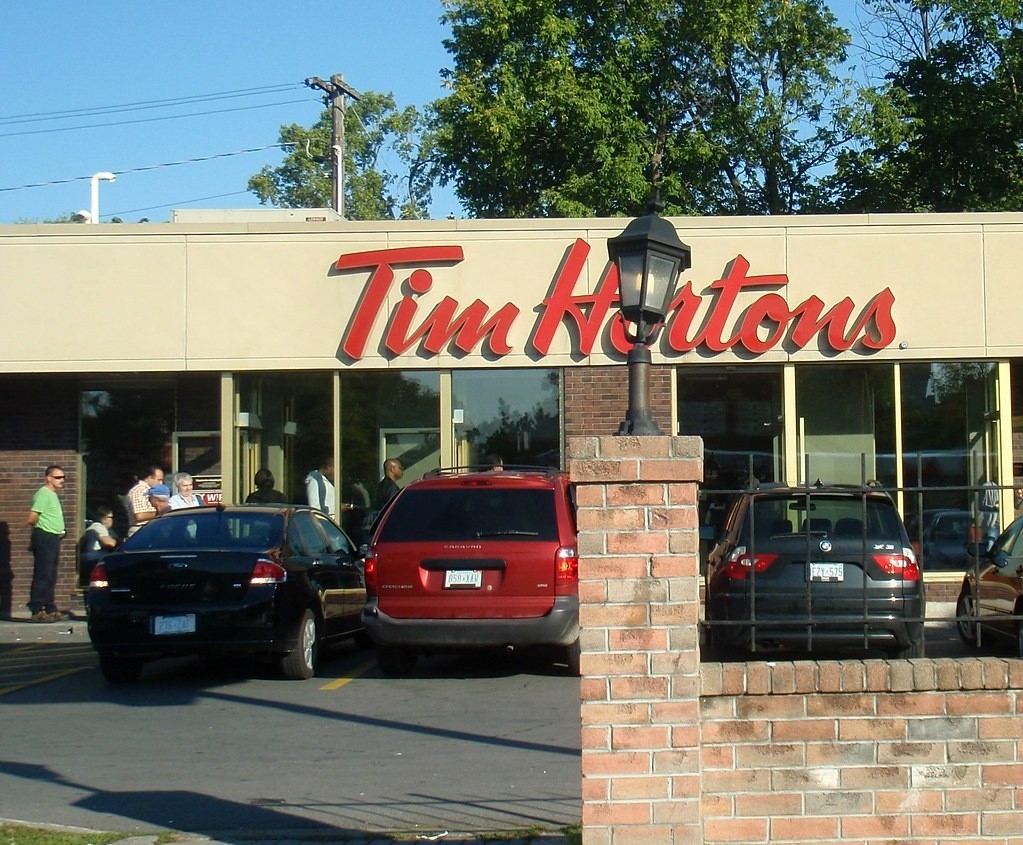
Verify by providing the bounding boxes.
[30,611,55,622]
[46,610,70,622]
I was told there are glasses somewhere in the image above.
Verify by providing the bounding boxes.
[107,515,114,519]
[50,475,66,480]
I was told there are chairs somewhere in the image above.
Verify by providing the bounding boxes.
[152,514,869,550]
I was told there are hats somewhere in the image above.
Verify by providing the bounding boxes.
[142,484,171,495]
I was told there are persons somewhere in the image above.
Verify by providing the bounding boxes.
[703,493,729,556]
[246,469,287,504]
[347,462,373,544]
[26,466,70,623]
[80,503,117,585]
[376,457,403,513]
[980,479,999,553]
[125,465,164,538]
[480,453,504,474]
[305,452,353,526]
[143,484,173,518]
[167,471,209,513]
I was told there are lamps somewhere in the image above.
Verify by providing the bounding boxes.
[608,186,692,436]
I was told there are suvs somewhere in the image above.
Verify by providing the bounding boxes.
[365,465,583,680]
[704,476,929,659]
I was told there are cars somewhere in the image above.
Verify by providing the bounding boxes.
[85,501,367,684]
[957,517,1023,655]
[925,512,976,567]
